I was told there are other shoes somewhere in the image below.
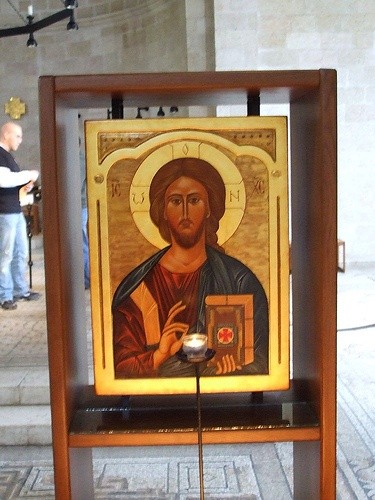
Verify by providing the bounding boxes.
[14,292,42,302]
[0,300,17,310]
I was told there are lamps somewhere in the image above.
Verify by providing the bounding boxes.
[0,0,79,49]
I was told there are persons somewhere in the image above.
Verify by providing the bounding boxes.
[0,122,40,311]
[76,134,89,290]
